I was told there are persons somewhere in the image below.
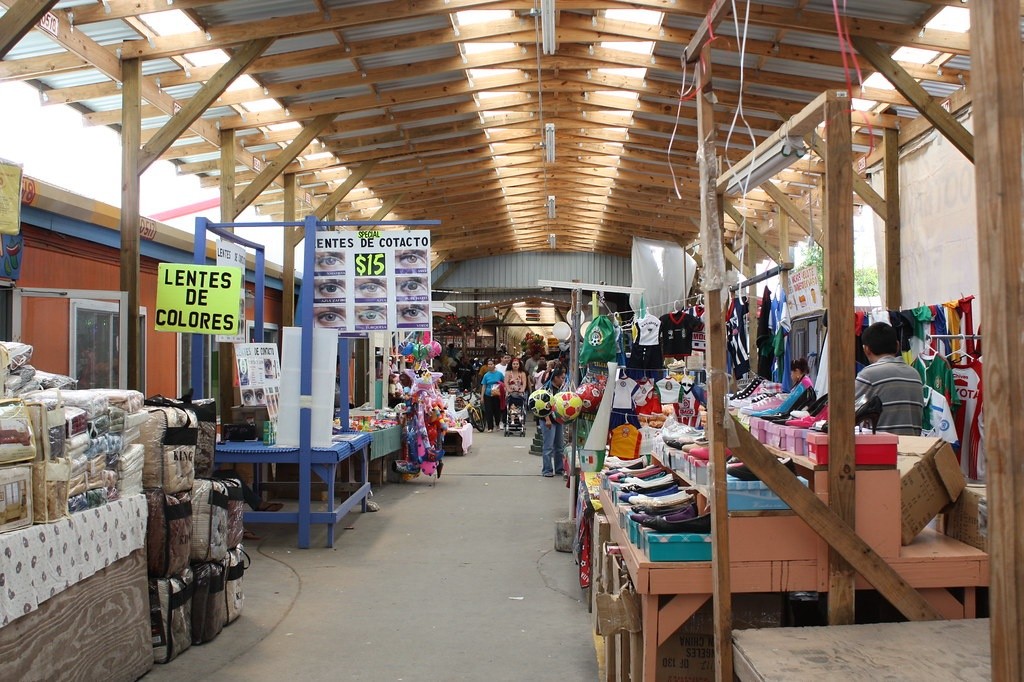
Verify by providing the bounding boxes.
[212,469,285,539]
[790,357,813,394]
[854,321,924,435]
[314,249,429,327]
[446,343,570,476]
[241,358,278,416]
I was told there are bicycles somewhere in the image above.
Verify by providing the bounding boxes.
[457,391,485,432]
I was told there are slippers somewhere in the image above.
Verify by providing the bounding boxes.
[244,531,262,541]
[256,502,284,511]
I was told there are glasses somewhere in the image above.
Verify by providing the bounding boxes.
[487,358,495,362]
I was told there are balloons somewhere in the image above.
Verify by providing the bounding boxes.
[553,306,592,343]
[521,331,545,356]
[397,339,442,363]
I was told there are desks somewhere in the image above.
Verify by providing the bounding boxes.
[280,413,411,488]
[199,428,371,550]
[600,442,992,682]
[0,494,158,682]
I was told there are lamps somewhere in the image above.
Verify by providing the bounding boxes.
[550,233,556,249]
[726,136,806,195]
[541,0,555,54]
[552,308,592,351]
[548,195,556,218]
[545,123,555,163]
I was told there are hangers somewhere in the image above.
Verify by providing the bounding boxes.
[595,268,789,387]
[910,334,1024,371]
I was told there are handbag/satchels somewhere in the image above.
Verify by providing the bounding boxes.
[579,314,615,366]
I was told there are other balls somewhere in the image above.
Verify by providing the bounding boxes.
[399,341,443,360]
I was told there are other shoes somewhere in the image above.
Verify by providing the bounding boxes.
[555,468,567,476]
[499,422,504,430]
[487,428,493,433]
[543,470,555,477]
[604,375,884,533]
[496,426,500,431]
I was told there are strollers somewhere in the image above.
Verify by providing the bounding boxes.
[504,391,526,437]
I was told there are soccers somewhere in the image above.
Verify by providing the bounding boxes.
[528,383,604,421]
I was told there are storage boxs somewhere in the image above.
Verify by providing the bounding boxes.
[590,407,1024,682]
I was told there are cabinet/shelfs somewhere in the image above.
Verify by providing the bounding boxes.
[394,327,467,412]
